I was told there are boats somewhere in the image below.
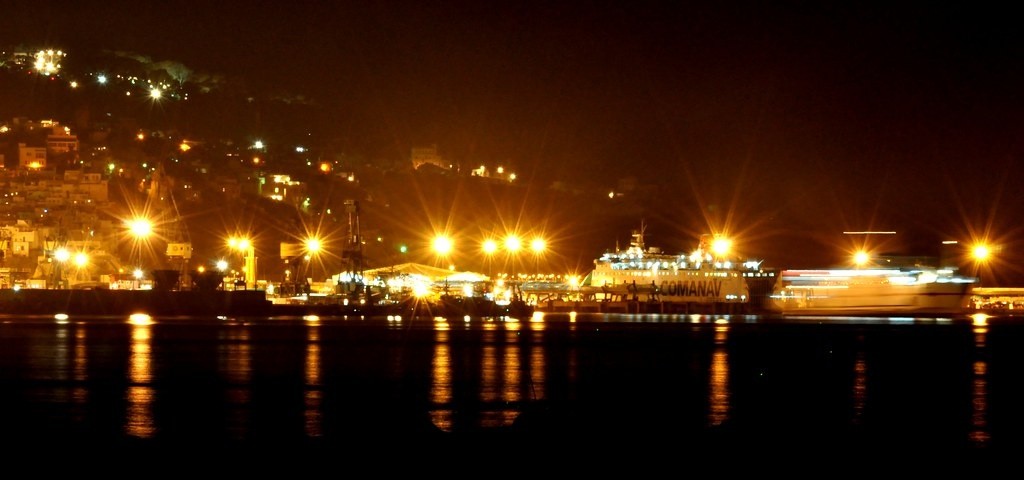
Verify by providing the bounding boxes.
[592,232,781,308]
[775,268,979,317]
